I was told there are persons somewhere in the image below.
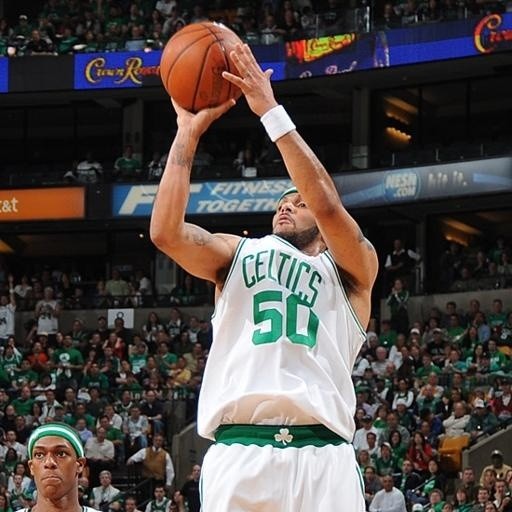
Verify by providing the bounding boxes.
[148,41,381,512]
[1,237,512,512]
[61,143,261,183]
[1,1,511,60]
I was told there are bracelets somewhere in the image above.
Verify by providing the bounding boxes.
[260,105,296,142]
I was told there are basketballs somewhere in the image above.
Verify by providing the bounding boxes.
[161,21,246,113]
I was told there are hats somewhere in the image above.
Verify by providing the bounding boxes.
[410,327,441,335]
[381,442,391,451]
[490,449,503,458]
[397,399,405,405]
[361,415,371,421]
